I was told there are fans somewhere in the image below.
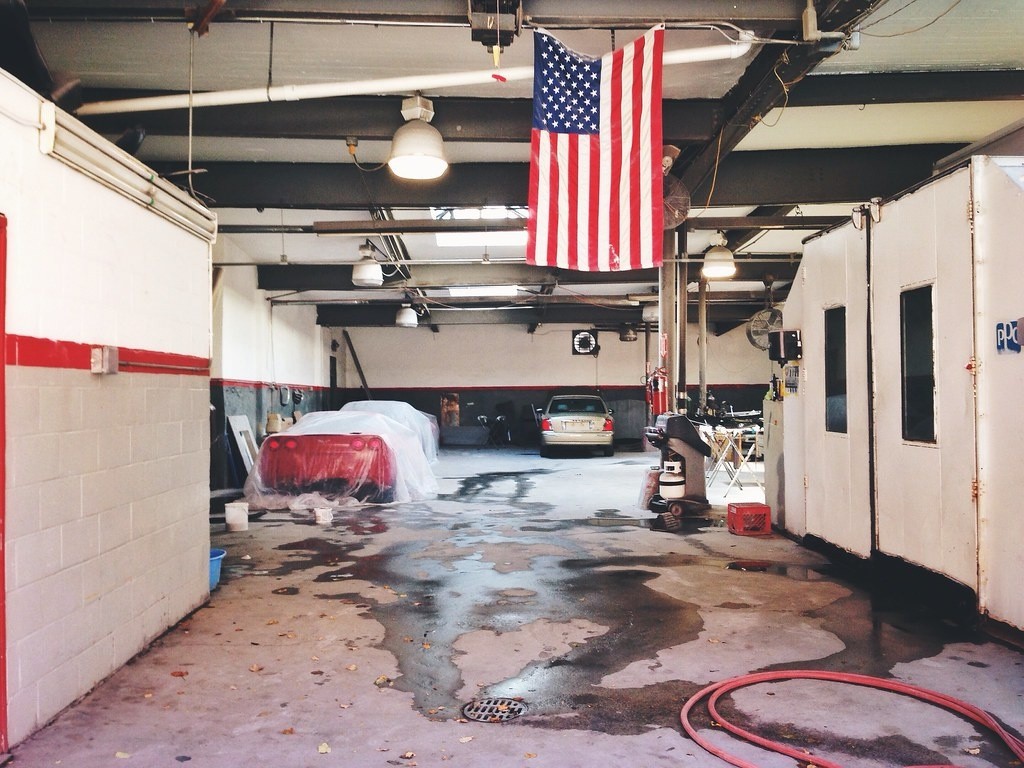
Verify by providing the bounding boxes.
[659,145,692,231]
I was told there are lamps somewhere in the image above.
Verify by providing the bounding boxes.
[701,245,739,279]
[352,246,384,286]
[390,97,449,179]
[397,304,418,327]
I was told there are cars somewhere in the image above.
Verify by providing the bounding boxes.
[258,401,440,503]
[536,394,616,458]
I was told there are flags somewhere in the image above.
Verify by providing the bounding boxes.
[525,23,667,273]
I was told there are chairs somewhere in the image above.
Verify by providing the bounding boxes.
[586,406,597,412]
[558,404,569,411]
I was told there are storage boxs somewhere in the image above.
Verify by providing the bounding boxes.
[728,503,773,535]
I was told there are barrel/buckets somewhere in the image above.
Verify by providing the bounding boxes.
[209,548,226,590]
[224,502,249,531]
[314,507,333,525]
